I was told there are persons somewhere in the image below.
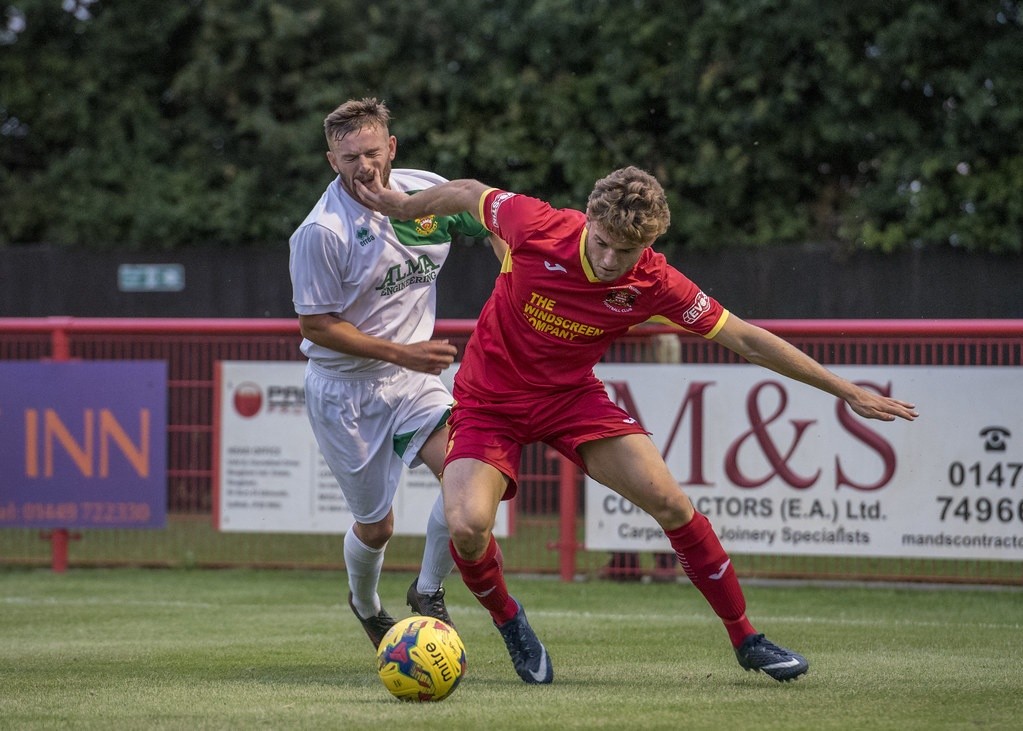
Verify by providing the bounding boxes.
[355,166,919,683]
[287,99,510,653]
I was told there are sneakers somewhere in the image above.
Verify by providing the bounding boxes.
[405,579,453,629]
[491,593,553,685]
[347,589,397,651]
[732,633,810,682]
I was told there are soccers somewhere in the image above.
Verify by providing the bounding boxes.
[377,618,466,699]
